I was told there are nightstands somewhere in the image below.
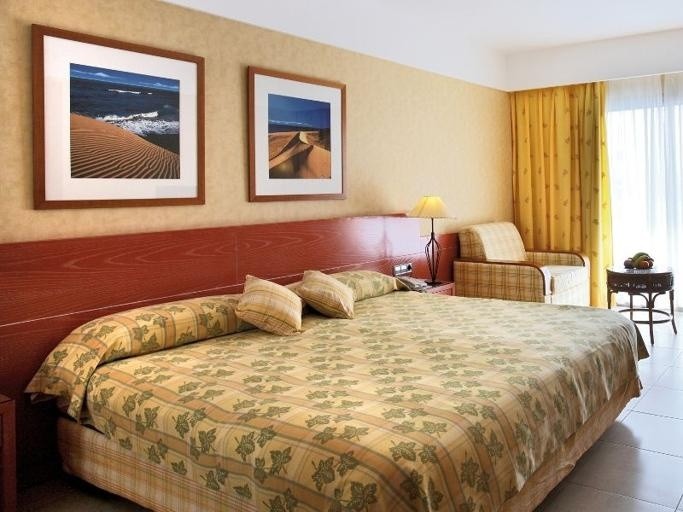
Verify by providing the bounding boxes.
[415,278,456,295]
[0,389,17,510]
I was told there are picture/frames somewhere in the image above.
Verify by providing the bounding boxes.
[246,65,349,202]
[28,22,207,211]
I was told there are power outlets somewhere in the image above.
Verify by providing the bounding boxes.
[394,263,412,275]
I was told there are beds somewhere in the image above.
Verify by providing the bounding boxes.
[0,214,649,512]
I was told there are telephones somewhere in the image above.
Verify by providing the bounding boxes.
[398,275,428,293]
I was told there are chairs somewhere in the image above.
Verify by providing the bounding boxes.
[453,221,591,306]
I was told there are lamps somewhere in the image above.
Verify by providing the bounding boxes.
[404,196,458,286]
